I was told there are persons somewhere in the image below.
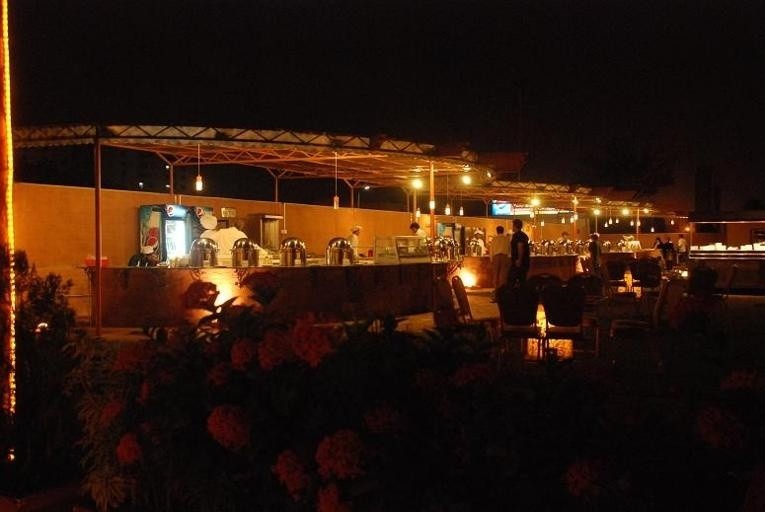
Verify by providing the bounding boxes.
[654,238,663,248]
[676,234,688,255]
[198,214,219,240]
[490,226,510,297]
[588,233,602,276]
[128,247,158,267]
[410,223,428,240]
[347,224,362,256]
[210,219,270,261]
[557,232,571,242]
[506,218,530,288]
[663,236,675,270]
[470,230,488,254]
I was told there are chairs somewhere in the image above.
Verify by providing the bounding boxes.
[435,258,682,370]
